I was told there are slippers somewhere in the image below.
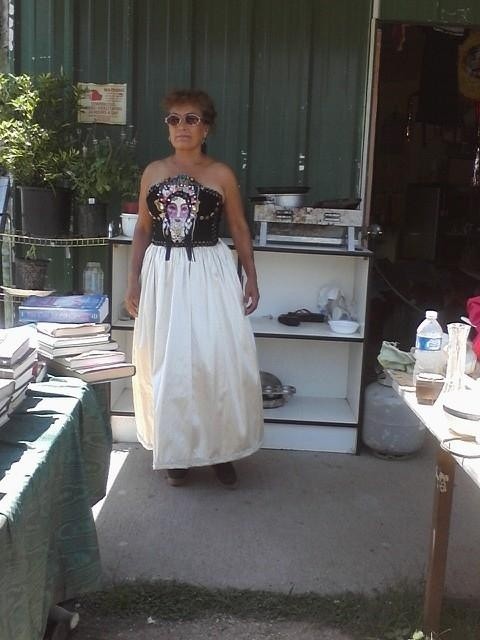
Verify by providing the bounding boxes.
[277,308,325,327]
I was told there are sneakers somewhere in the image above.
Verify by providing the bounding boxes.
[167,469,189,486]
[213,462,238,489]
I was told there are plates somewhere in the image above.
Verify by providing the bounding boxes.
[317,198,362,210]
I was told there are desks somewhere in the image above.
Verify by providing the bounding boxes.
[0,351,112,640]
[378,347,480,638]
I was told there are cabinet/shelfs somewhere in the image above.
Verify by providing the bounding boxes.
[0,220,375,463]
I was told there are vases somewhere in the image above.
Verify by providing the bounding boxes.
[14,254,49,291]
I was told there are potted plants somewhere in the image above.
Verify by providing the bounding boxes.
[1,66,143,239]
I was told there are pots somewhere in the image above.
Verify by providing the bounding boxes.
[244,185,310,209]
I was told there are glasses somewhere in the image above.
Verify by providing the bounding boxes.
[164,112,207,126]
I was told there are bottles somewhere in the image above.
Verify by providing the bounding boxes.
[444,323,469,395]
[413,310,443,386]
[81,261,104,295]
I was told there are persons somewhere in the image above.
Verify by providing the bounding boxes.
[123,88,259,489]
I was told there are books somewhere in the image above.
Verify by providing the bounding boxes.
[0,294,136,427]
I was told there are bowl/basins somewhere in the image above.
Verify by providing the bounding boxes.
[328,320,359,334]
